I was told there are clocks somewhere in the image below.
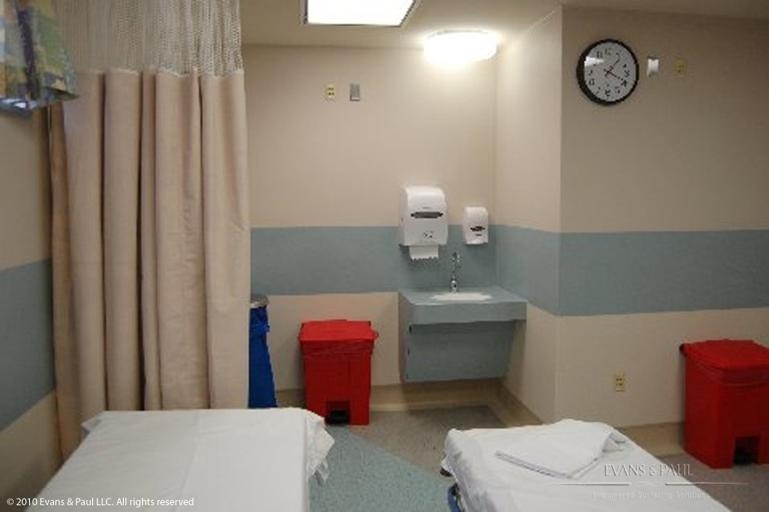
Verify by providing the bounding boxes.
[576,38,639,106]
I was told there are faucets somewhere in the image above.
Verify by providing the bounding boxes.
[449,249,461,293]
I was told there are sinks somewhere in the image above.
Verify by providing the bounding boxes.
[433,291,490,301]
